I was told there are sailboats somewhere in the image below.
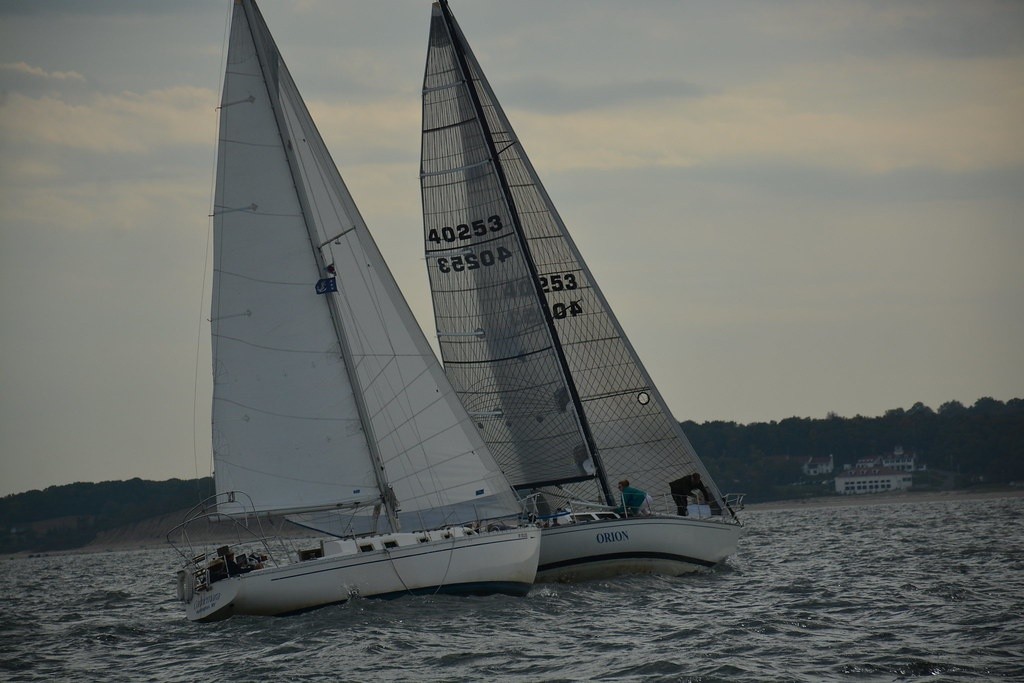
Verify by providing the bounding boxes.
[420,0,744,577]
[166,1,541,624]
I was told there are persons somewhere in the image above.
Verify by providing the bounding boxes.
[668,473,709,516]
[617,479,647,519]
[225,550,263,577]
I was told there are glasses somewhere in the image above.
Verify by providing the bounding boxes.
[618,486,622,489]
